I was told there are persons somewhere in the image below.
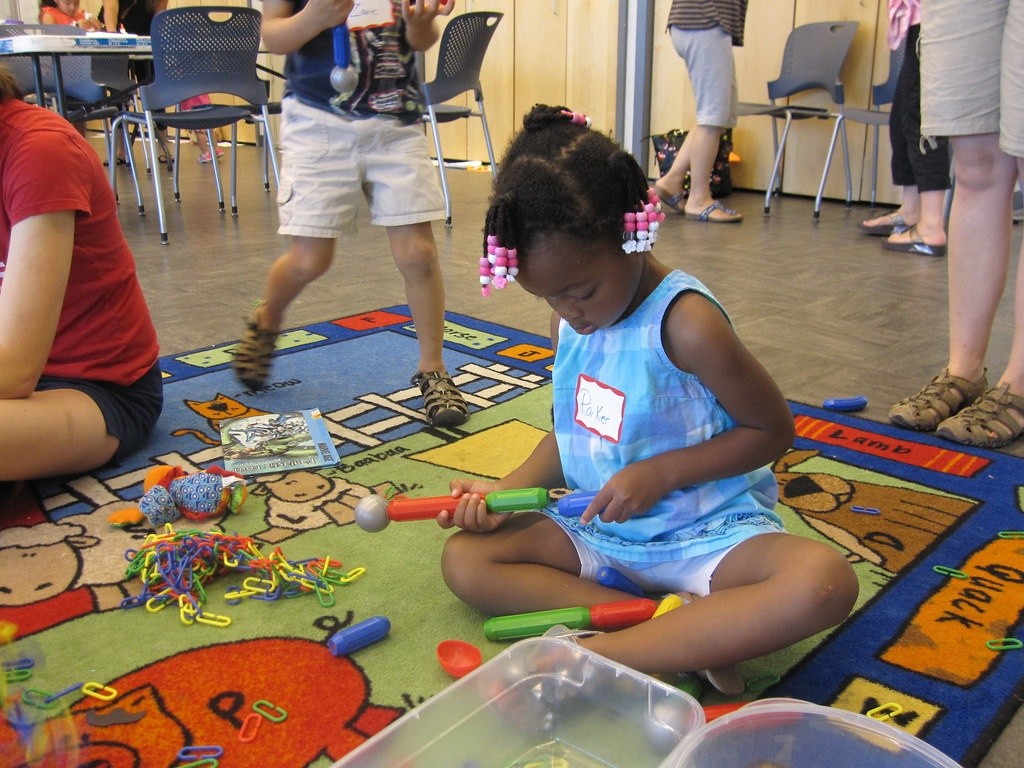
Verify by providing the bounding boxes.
[655,0,749,222]
[437,106,860,731]
[180,94,224,162]
[39,0,102,135]
[888,0,1024,447]
[856,0,953,254]
[235,0,470,427]
[0,63,164,482]
[103,0,174,166]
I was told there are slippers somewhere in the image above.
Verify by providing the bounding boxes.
[653,185,687,216]
[102,157,127,166]
[157,153,174,163]
[684,199,743,224]
[880,223,947,257]
[857,206,914,237]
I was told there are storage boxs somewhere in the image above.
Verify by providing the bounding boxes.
[659,696,963,768]
[330,637,706,766]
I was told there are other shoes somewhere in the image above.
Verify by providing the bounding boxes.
[215,147,224,158]
[198,151,213,164]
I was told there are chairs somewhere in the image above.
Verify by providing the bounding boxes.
[417,10,505,226]
[814,36,907,218]
[107,4,283,243]
[717,22,857,214]
[1,24,146,218]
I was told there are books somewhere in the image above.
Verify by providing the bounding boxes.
[219,407,341,476]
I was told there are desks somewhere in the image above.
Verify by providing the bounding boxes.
[0,37,281,184]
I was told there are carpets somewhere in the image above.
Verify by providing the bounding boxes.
[0,304,1023,768]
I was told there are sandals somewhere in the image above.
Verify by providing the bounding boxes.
[232,298,280,391]
[411,368,471,428]
[887,365,990,431]
[933,380,1024,449]
